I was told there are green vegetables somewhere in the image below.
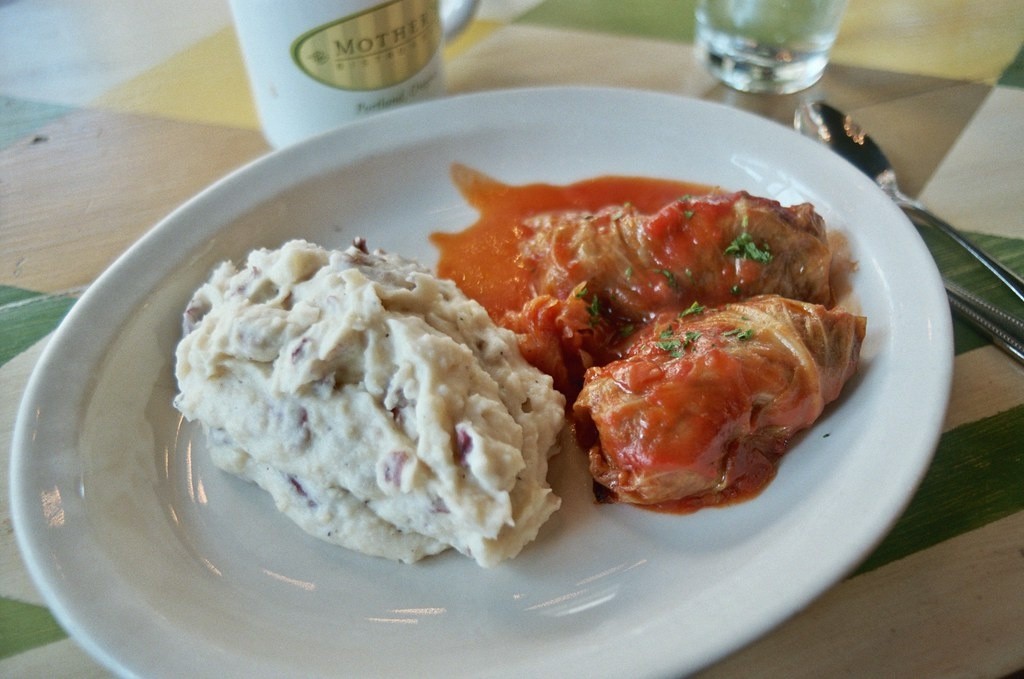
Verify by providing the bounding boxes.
[573,194,775,360]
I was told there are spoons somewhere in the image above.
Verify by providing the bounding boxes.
[794,102,1024,303]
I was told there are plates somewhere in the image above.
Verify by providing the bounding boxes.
[9,82,956,679]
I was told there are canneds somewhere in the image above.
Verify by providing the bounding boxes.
[227,0,441,152]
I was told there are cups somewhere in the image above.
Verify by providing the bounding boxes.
[691,0,849,96]
[229,0,481,150]
